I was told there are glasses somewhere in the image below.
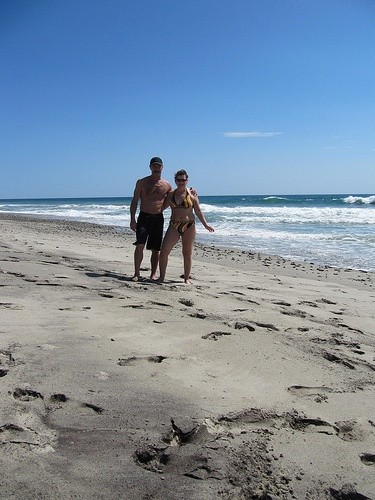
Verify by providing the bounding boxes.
[176,178,187,182]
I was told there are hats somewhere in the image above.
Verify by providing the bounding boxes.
[150,157,162,165]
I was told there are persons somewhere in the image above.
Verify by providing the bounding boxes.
[154,169,215,284]
[129,157,198,282]
